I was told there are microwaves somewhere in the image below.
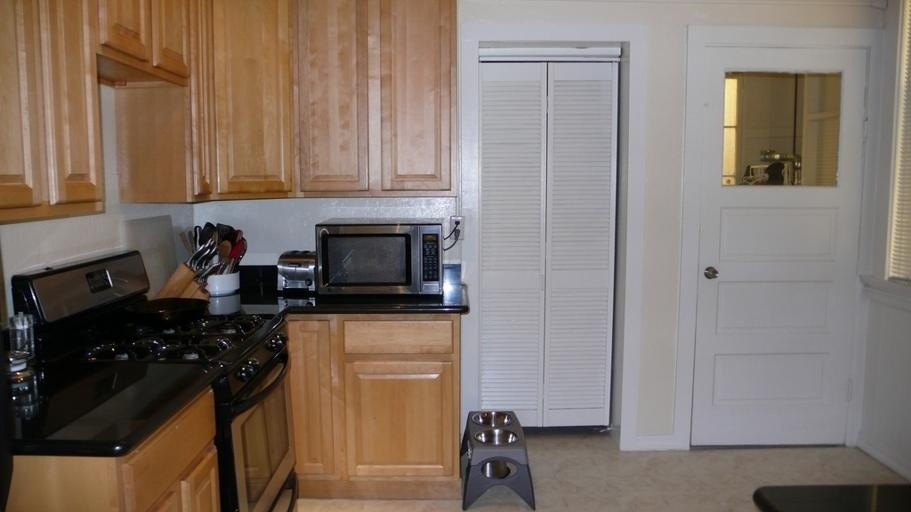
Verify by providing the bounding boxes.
[314,217,442,297]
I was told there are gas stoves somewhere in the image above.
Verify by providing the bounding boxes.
[88,309,288,401]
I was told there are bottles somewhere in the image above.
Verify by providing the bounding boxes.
[11,360,39,420]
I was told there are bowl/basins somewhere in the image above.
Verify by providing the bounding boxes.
[469,411,519,444]
[205,272,240,296]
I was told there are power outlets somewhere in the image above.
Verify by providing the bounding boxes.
[449,216,465,241]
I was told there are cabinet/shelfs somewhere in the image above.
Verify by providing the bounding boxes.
[118,2,214,205]
[120,382,222,512]
[214,0,295,201]
[92,0,188,90]
[0,1,106,226]
[294,1,458,200]
[285,309,461,501]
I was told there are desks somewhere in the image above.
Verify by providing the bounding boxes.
[753,483,910,512]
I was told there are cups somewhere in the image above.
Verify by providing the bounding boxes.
[10,311,36,360]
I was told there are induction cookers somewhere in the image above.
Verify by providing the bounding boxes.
[11,248,150,341]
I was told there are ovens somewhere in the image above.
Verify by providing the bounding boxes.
[218,351,295,512]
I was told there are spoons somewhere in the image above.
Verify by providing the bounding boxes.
[217,238,248,275]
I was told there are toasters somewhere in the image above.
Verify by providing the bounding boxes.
[277,251,316,292]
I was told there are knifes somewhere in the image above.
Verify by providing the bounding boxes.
[186,238,220,284]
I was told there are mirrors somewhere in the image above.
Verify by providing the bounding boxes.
[720,70,843,189]
